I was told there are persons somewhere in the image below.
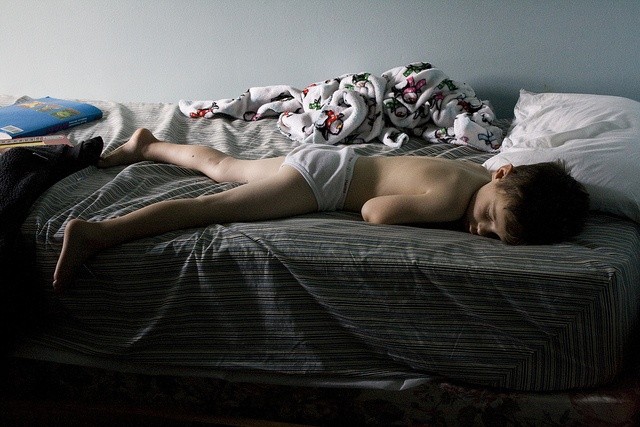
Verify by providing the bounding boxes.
[52,126,593,292]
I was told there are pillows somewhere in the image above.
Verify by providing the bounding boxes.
[481,87,640,223]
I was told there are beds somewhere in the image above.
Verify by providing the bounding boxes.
[1,98,640,427]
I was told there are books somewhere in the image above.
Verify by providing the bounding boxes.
[0,94,105,142]
[0,133,74,156]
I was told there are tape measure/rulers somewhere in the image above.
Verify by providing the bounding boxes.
[0,137,43,143]
[2,135,66,140]
